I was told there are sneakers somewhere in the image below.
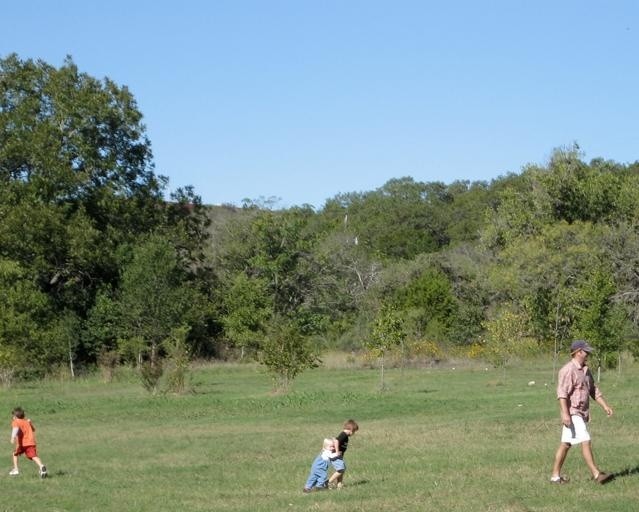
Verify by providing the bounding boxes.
[10,469,20,476]
[39,465,47,478]
[303,482,345,493]
[550,477,568,485]
[594,472,615,485]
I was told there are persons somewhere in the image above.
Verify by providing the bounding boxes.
[299,437,343,491]
[6,406,47,479]
[322,419,360,490]
[548,339,616,485]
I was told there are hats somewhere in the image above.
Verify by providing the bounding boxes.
[570,340,592,356]
